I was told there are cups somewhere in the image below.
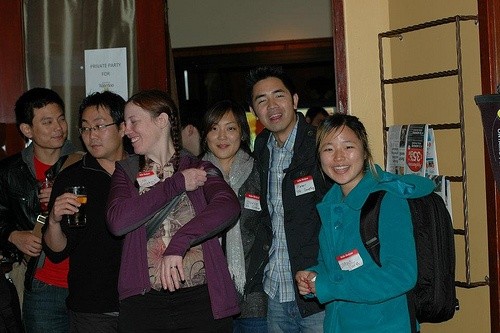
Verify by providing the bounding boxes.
[63,186,87,227]
[37,175,56,214]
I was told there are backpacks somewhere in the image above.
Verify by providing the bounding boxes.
[358,188,460,333]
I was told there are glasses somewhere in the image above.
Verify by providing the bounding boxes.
[78,122,116,137]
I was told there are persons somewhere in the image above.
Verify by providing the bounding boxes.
[41,91,136,333]
[306,106,329,129]
[0,88,85,333]
[246,66,335,333]
[174,108,208,160]
[195,99,272,333]
[105,90,241,333]
[294,113,436,333]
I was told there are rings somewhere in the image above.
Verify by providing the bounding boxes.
[170,266,177,268]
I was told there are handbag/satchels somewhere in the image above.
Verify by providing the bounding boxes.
[8,260,28,318]
